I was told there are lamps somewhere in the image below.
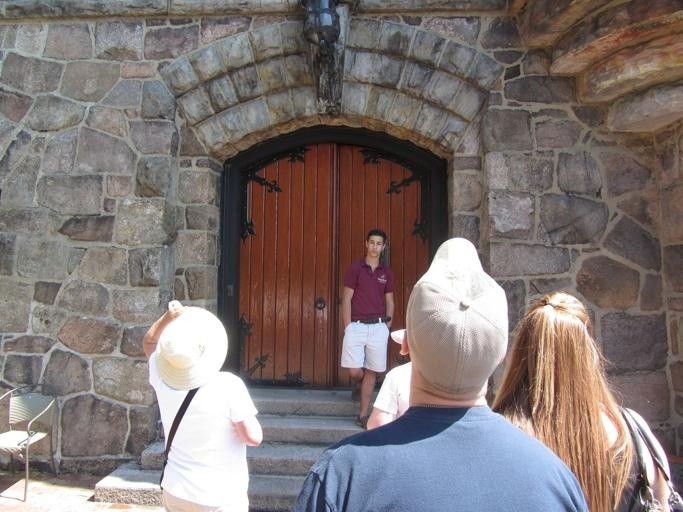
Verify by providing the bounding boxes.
[301,0,340,54]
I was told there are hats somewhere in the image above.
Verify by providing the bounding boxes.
[401,236,511,395]
[151,305,232,393]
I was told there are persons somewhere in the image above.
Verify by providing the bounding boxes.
[364,326,412,430]
[340,229,395,428]
[491,291,683,510]
[142,299,263,511]
[289,237,587,511]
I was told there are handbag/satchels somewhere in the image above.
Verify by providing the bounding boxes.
[639,486,683,512]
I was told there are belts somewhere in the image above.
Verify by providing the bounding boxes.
[349,313,392,325]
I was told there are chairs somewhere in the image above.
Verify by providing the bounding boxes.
[0,383,59,502]
